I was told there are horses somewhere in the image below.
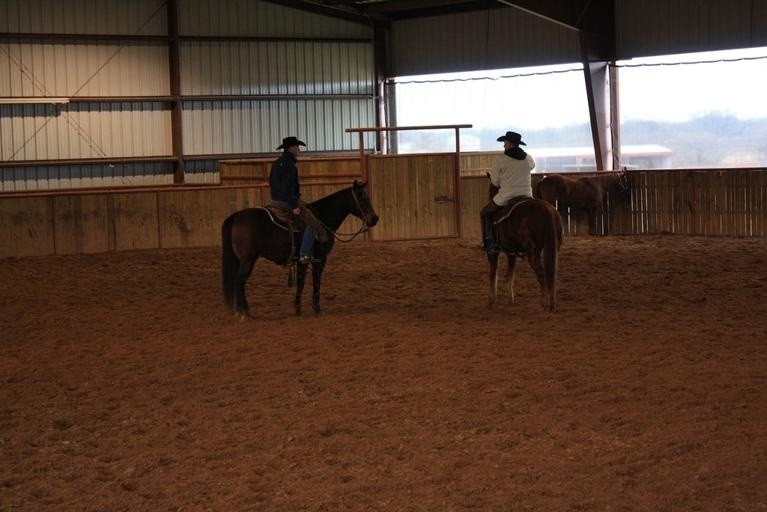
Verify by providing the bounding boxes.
[219,179,380,322]
[477,169,565,315]
[536,166,630,238]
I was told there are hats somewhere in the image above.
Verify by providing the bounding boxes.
[277,137,306,150]
[498,132,526,147]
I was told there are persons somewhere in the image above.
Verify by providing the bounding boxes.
[267,136,323,267]
[478,130,536,251]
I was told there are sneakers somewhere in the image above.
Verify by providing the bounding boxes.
[299,254,319,265]
